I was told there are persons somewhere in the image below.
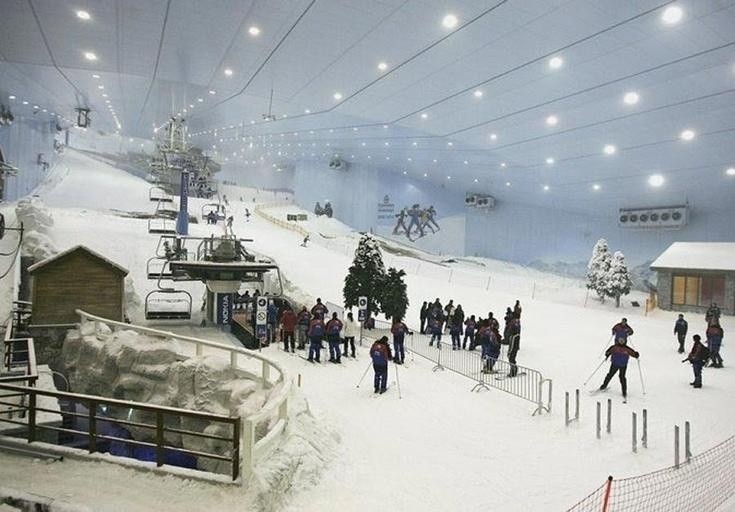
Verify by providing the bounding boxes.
[674,314,687,353]
[709,302,720,317]
[234,290,521,378]
[213,211,218,224]
[370,336,394,394]
[613,318,634,345]
[705,316,723,368]
[301,234,310,247]
[207,211,215,224]
[599,333,639,398]
[688,334,706,388]
[392,204,440,237]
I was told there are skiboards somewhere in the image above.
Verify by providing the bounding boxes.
[342,351,358,361]
[299,354,322,363]
[481,368,505,374]
[495,371,526,380]
[588,385,628,403]
[368,381,393,398]
